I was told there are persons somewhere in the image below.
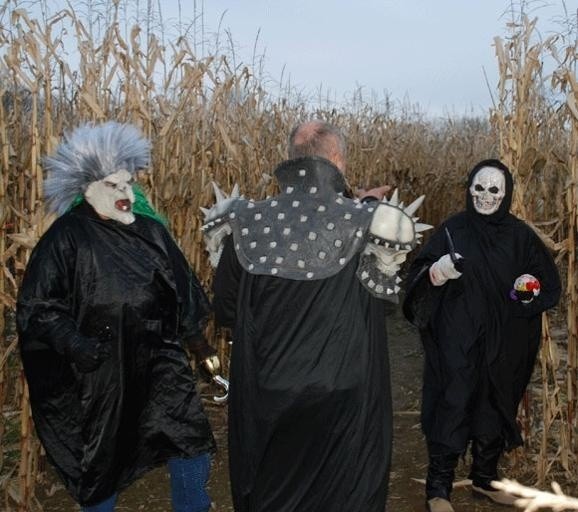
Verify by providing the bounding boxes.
[403,158,561,511]
[199,119,434,512]
[15,122,230,512]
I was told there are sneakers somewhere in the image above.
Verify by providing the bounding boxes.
[427,497,454,512]
[471,485,519,505]
